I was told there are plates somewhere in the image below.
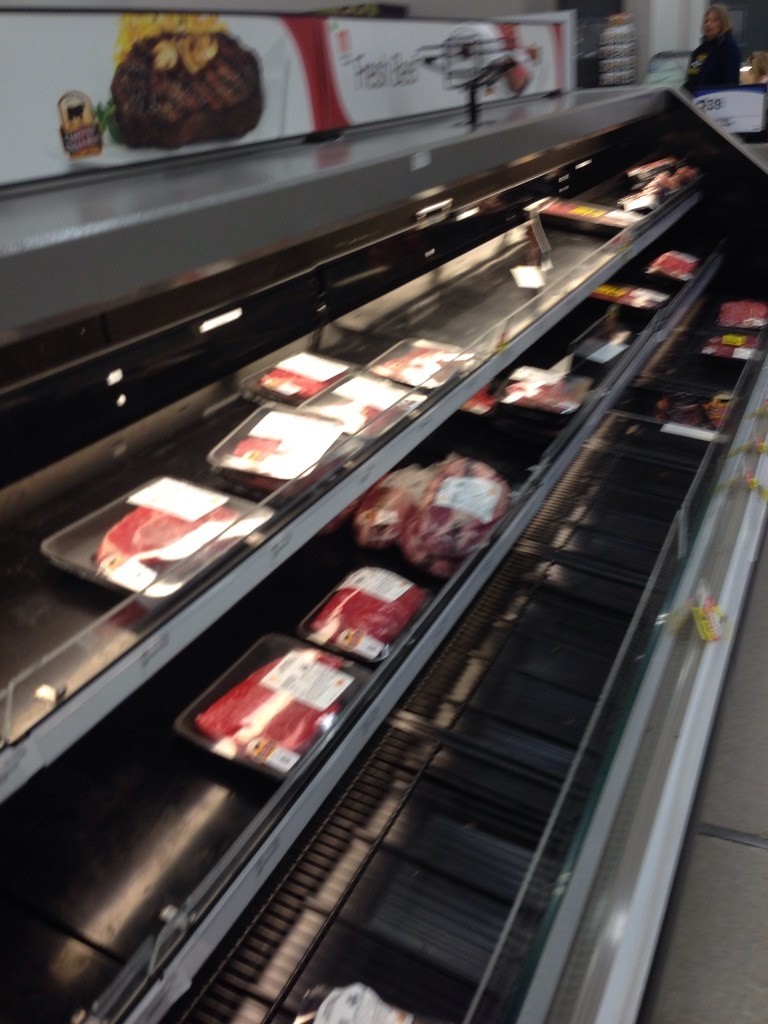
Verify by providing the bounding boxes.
[175,633,373,780]
[366,337,475,389]
[41,475,274,609]
[205,351,430,497]
[299,566,435,661]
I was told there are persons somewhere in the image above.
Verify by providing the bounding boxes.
[679,6,741,99]
[746,50,768,85]
